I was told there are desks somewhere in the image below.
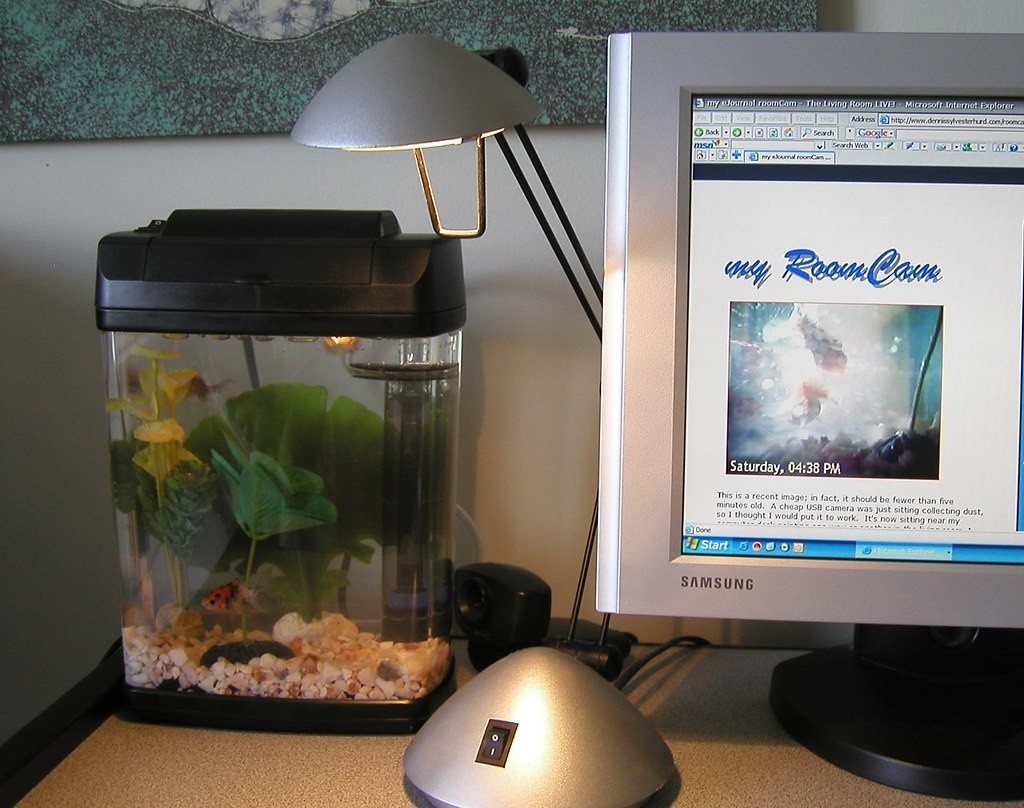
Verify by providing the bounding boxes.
[0,642,1024,808]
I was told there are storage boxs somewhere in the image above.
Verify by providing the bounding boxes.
[95,209,471,737]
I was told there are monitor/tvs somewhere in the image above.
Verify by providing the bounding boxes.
[595,29,1024,802]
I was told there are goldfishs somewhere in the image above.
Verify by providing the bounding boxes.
[200,576,266,614]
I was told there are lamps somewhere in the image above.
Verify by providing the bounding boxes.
[290,34,674,808]
